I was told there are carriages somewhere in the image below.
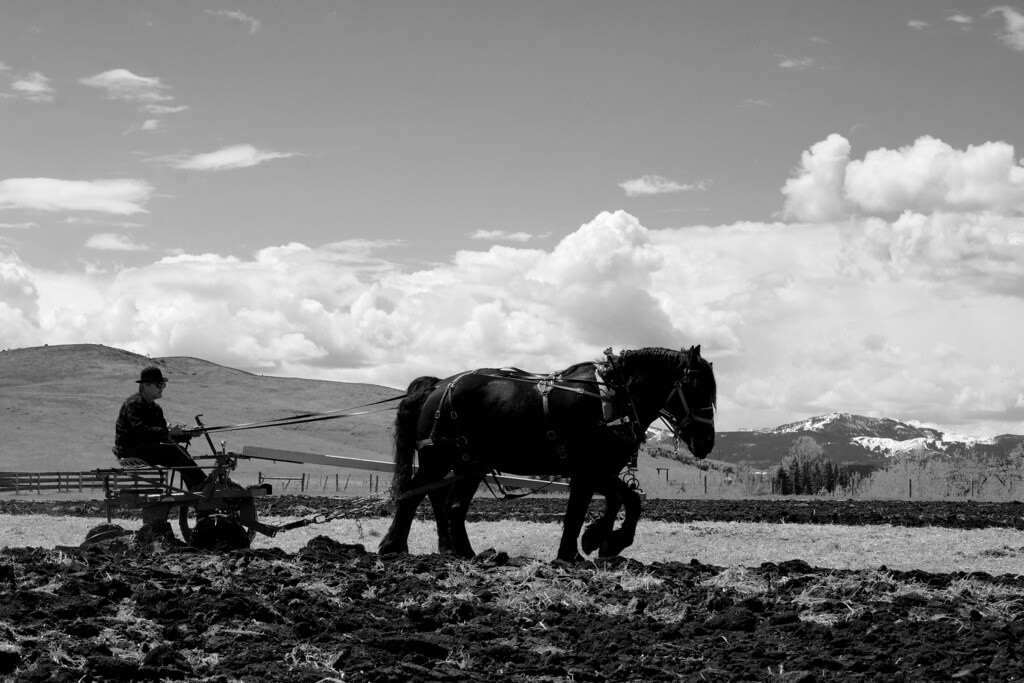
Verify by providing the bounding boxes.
[77,343,720,567]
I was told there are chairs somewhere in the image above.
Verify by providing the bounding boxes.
[112,445,152,467]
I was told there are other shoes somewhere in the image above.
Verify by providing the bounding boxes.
[190,477,210,492]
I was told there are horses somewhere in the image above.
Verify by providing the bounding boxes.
[375,343,718,567]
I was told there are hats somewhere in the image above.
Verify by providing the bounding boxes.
[135,367,168,383]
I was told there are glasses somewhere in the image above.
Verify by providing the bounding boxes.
[151,382,167,388]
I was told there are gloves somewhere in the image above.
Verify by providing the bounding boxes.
[170,423,185,436]
[190,425,205,437]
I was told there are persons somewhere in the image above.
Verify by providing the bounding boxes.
[115,367,210,492]
